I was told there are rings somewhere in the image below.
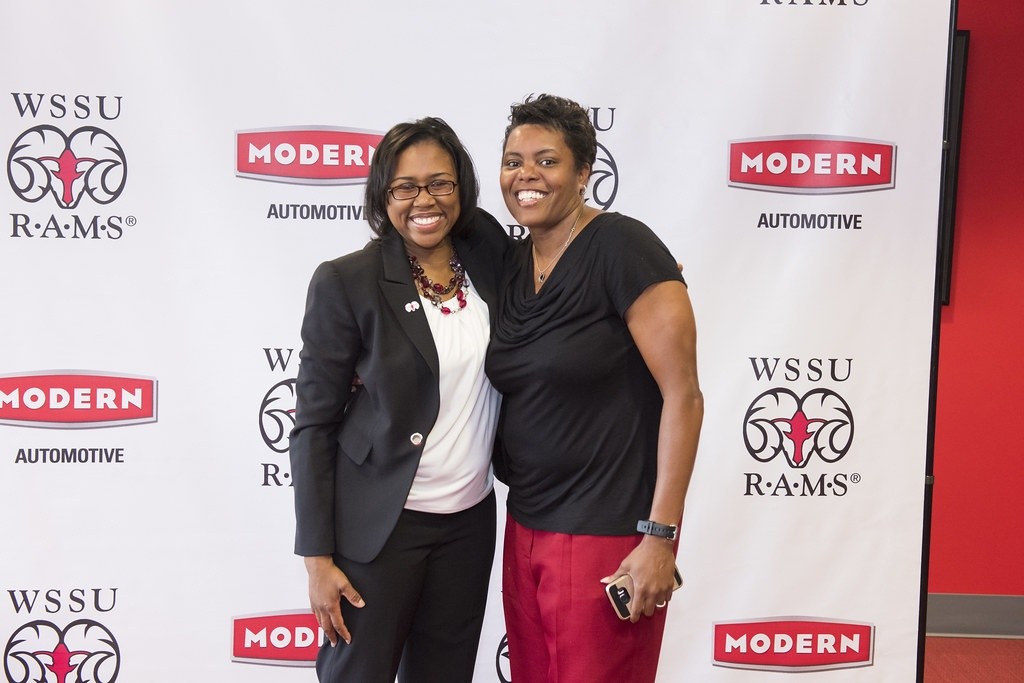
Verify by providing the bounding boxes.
[655,599,667,609]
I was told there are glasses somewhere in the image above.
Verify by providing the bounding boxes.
[387,179,458,200]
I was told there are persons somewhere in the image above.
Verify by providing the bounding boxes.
[483,96,705,683]
[289,118,517,683]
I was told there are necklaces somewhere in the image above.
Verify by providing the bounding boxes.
[406,242,469,316]
[520,205,583,283]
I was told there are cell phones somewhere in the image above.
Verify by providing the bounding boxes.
[606,563,683,620]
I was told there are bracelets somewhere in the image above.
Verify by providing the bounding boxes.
[636,519,681,542]
